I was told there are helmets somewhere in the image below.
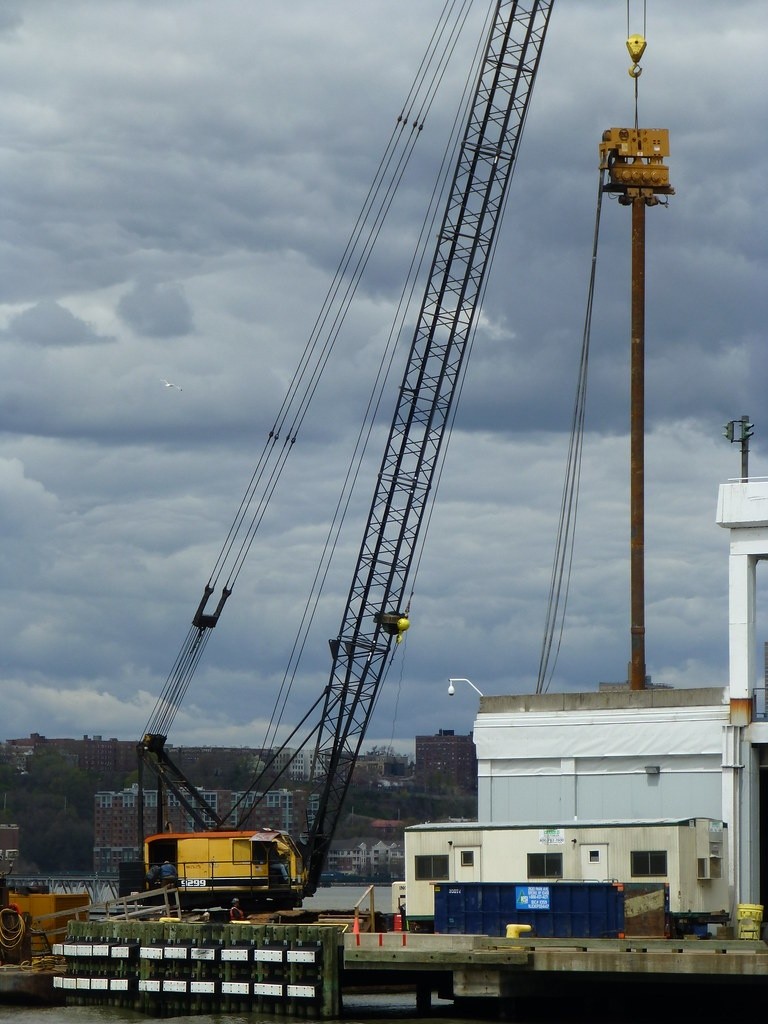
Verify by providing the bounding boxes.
[231,898,239,903]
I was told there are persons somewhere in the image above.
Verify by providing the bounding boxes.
[267,841,291,884]
[230,898,245,922]
[157,861,177,888]
[145,864,161,892]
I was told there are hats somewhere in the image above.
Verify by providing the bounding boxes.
[164,861,169,863]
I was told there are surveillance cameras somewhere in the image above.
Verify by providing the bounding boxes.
[448,685,455,695]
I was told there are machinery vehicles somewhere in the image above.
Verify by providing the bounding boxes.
[118,0,679,920]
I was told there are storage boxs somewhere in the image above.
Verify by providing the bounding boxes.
[717,927,734,936]
